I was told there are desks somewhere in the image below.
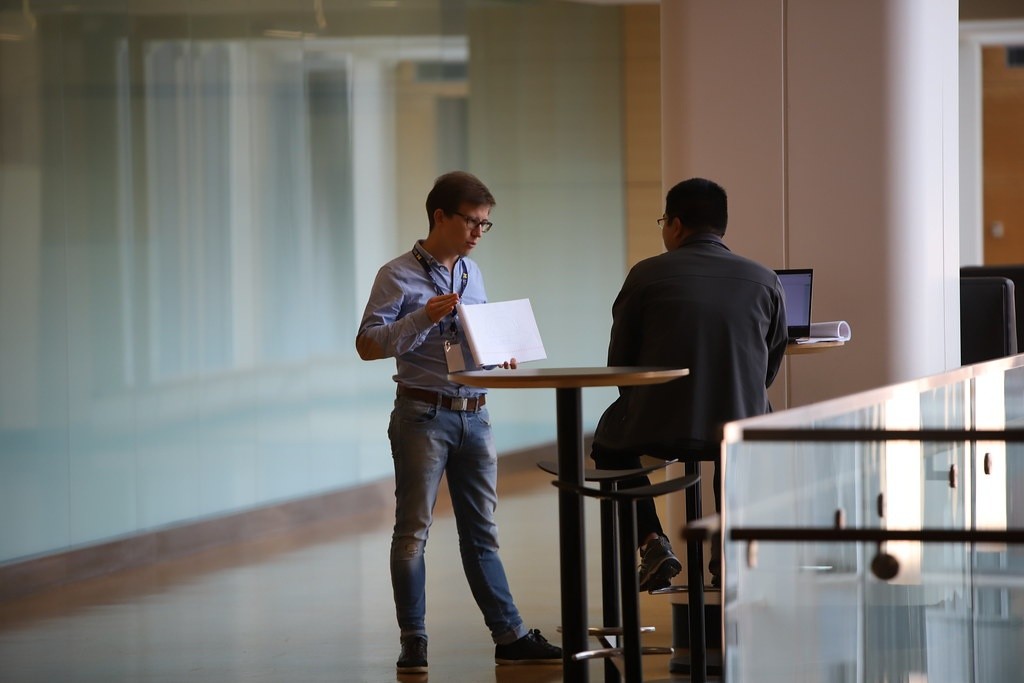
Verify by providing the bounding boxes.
[783,340,847,356]
[446,364,691,682]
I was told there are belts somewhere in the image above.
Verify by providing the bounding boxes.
[397,387,486,412]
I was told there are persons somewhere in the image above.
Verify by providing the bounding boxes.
[589,177,787,593]
[355,171,563,672]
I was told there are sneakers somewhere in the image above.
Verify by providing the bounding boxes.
[494,633,563,663]
[638,537,681,590]
[395,636,430,673]
[709,567,721,586]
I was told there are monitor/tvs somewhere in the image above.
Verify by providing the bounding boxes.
[772,268,814,338]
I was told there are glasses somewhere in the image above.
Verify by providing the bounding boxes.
[657,216,677,229]
[442,209,493,233]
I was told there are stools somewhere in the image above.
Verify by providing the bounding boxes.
[527,452,681,682]
[629,439,726,683]
[550,475,707,683]
